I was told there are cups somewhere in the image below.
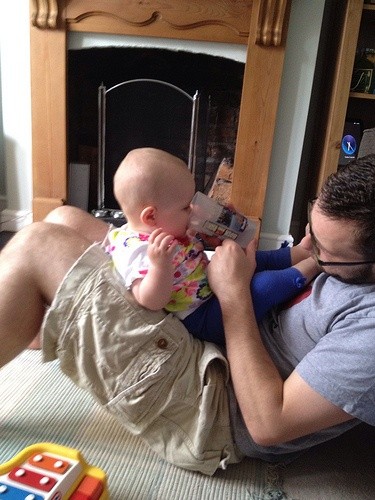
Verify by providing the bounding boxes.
[189,191,256,248]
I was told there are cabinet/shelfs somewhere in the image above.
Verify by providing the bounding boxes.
[289,0,375,247]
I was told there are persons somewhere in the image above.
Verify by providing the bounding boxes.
[0,159,375,478]
[107,147,324,346]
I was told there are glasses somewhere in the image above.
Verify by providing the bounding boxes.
[308,198,372,267]
